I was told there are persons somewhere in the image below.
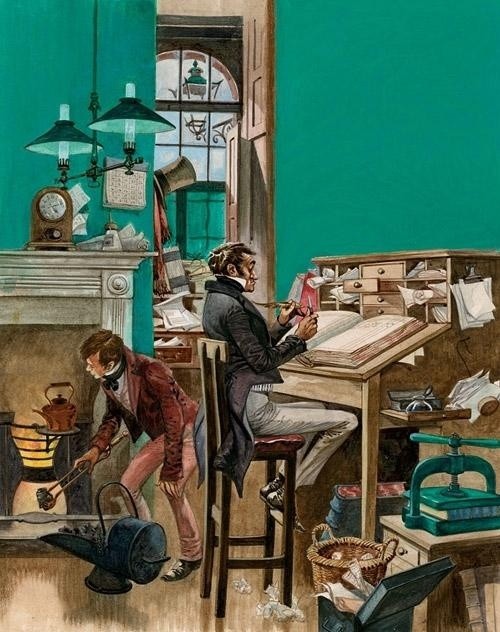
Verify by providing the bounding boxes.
[74,329,203,581]
[201,241,359,535]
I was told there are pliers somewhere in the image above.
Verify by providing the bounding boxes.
[37,429,128,511]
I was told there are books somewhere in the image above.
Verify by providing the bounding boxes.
[325,482,411,540]
[273,308,428,368]
[163,245,218,296]
[102,157,149,212]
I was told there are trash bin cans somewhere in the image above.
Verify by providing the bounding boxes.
[306,524,401,591]
